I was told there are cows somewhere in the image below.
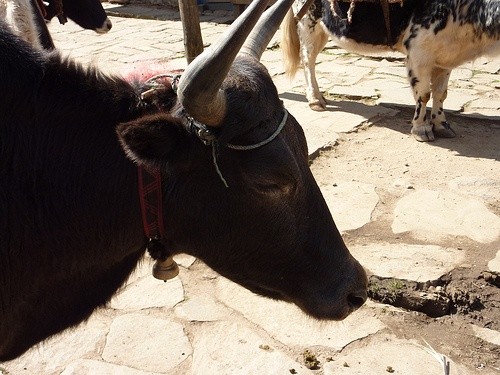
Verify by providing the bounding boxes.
[1,1,368,362]
[35,0,113,32]
[286,0,499,142]
